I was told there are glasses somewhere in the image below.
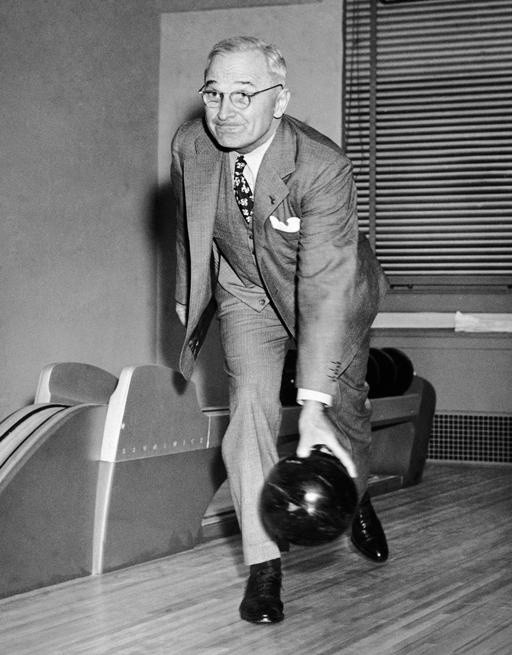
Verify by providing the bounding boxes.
[198,83,285,111]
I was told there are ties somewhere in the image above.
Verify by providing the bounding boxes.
[232,156,254,226]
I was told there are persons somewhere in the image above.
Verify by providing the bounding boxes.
[169,36,389,622]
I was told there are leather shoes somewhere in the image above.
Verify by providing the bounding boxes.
[351,496,388,564]
[240,558,284,626]
